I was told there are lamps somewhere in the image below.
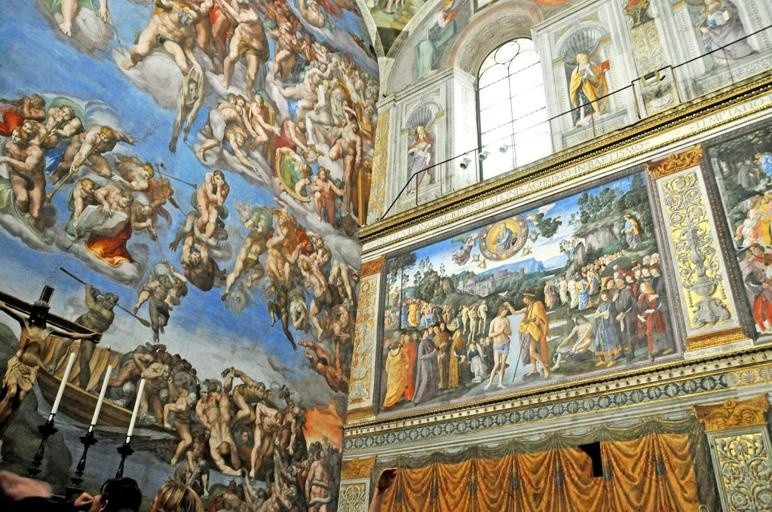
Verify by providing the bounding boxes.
[457,140,509,170]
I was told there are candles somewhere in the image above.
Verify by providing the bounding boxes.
[92,363,115,427]
[127,378,149,437]
[50,352,76,414]
